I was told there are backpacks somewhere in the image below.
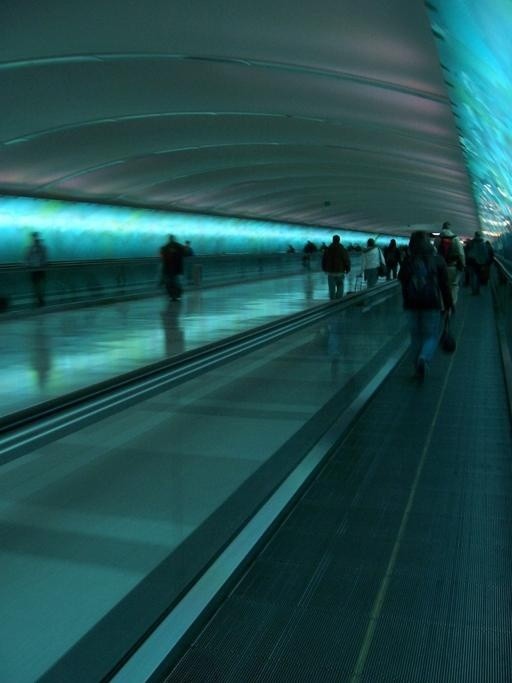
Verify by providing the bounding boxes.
[439,235,456,261]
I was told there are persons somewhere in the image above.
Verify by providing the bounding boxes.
[26,229,50,307]
[162,234,184,301]
[303,222,494,310]
[286,245,294,261]
[184,241,192,256]
[399,229,455,381]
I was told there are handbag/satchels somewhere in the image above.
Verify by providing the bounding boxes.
[440,330,456,354]
[378,264,388,278]
